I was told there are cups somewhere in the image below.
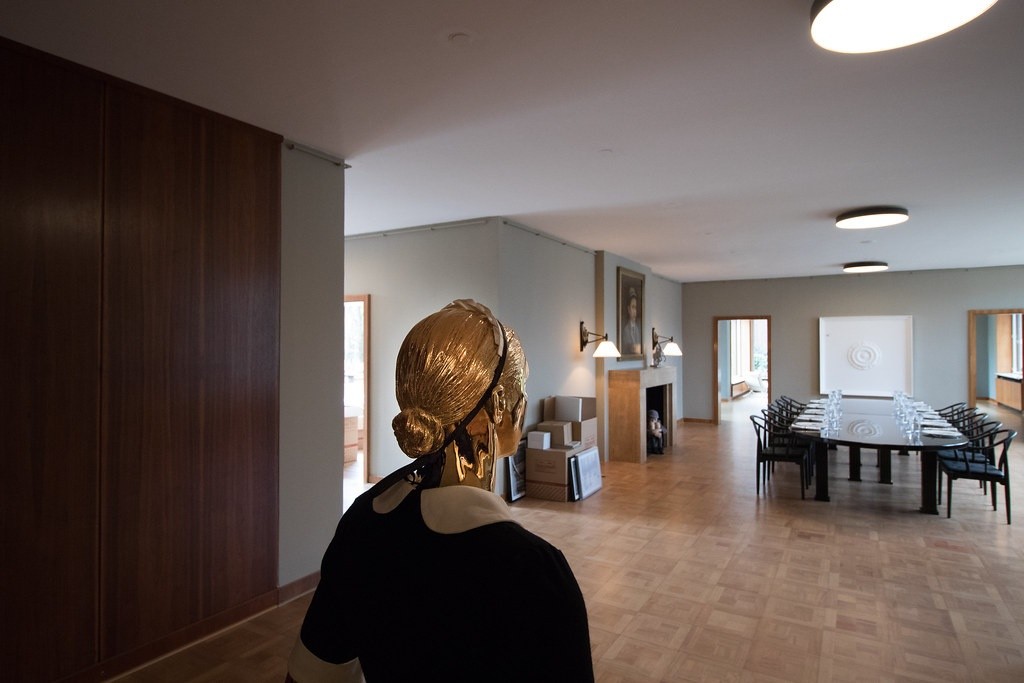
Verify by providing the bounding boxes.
[823,389,843,438]
[892,390,921,444]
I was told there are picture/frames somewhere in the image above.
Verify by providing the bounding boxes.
[507,438,526,502]
[617,266,646,361]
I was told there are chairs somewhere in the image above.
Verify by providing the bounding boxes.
[916,402,1018,525]
[750,395,816,500]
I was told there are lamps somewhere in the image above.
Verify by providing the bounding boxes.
[843,261,888,273]
[580,321,622,358]
[652,327,683,355]
[835,207,909,230]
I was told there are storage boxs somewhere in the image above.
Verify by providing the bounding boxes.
[526,395,598,503]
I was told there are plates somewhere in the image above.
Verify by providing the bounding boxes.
[913,402,924,407]
[797,414,823,421]
[921,419,952,427]
[916,406,935,412]
[921,412,941,419]
[809,399,823,403]
[807,404,823,408]
[791,421,822,430]
[922,427,963,438]
[804,409,823,414]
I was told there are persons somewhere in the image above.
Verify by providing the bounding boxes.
[623,287,641,351]
[284,297,598,682]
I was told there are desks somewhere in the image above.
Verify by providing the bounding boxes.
[788,400,970,515]
